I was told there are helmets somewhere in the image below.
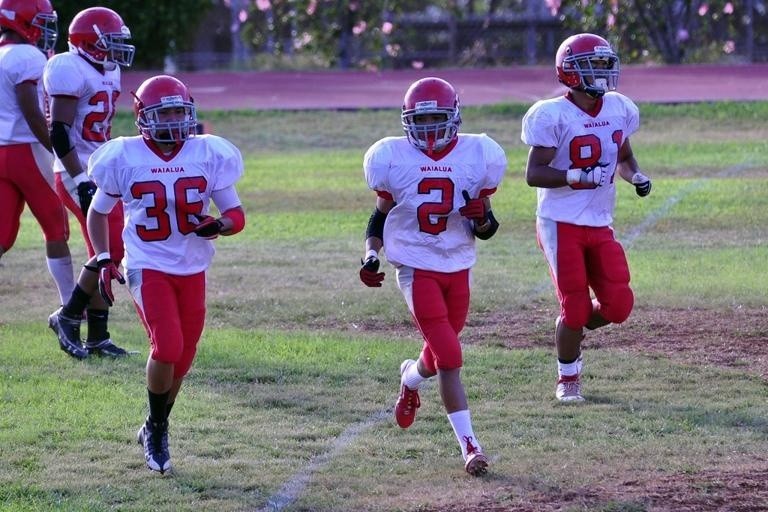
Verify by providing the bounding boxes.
[556,33,619,91]
[401,77,460,149]
[0,0,135,67]
[130,75,197,142]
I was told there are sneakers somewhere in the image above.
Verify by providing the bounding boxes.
[464,447,488,475]
[395,359,420,428]
[49,305,130,360]
[556,316,585,403]
[137,416,171,475]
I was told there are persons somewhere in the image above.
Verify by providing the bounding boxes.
[520,31,650,406]
[354,76,509,478]
[0,1,78,307]
[44,7,134,362]
[84,76,249,475]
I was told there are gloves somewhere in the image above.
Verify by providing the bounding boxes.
[188,213,225,240]
[97,253,125,306]
[566,166,606,190]
[72,172,98,217]
[459,190,486,225]
[632,172,651,197]
[360,256,385,287]
